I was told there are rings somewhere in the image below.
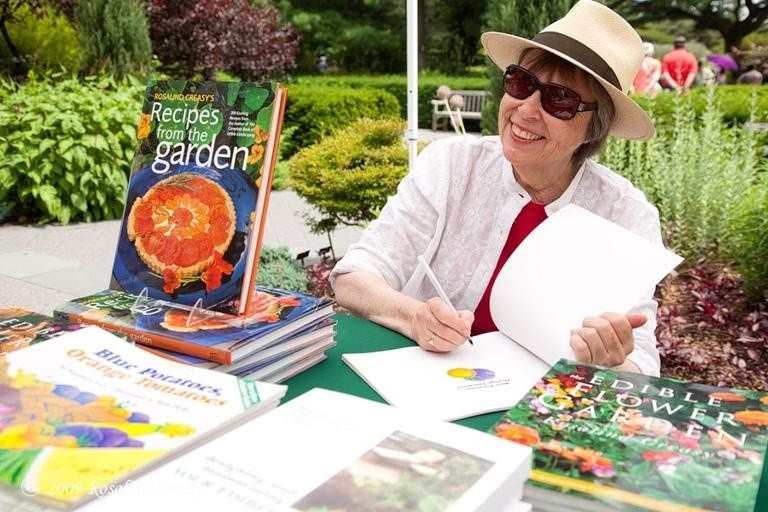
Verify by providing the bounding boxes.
[427,333,439,349]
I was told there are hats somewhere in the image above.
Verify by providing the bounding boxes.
[480,0,654,140]
[643,42,653,55]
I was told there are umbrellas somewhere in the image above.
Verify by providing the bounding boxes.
[707,53,738,71]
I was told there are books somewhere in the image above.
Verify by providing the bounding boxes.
[53,285,338,385]
[0,306,290,512]
[484,358,768,512]
[75,388,538,512]
[341,200,686,422]
[110,77,289,323]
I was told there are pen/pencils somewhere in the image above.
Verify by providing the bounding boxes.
[417,255,473,348]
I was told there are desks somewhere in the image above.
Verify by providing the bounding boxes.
[276,308,767,512]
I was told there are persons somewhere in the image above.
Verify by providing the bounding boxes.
[633,40,664,92]
[329,1,668,378]
[707,64,768,85]
[660,34,698,92]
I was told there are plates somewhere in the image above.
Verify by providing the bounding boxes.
[135,286,317,347]
[112,152,259,310]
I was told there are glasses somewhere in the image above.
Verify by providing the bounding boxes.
[503,64,598,119]
